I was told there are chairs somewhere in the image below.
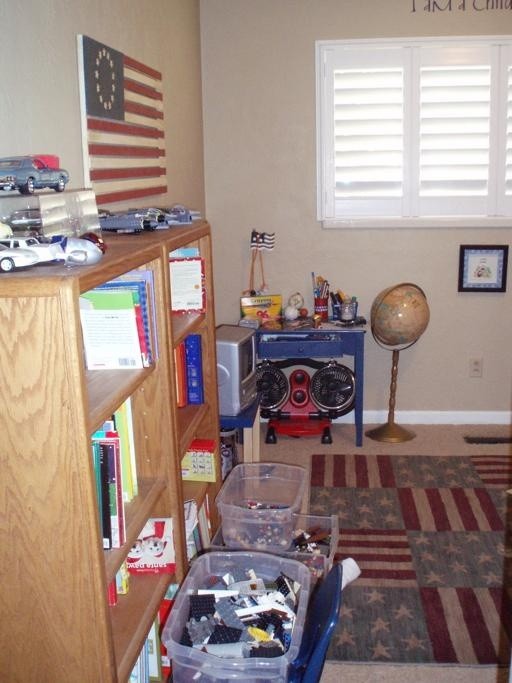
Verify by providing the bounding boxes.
[290,565,341,683]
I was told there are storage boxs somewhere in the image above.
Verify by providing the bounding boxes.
[161,551,312,683]
[209,514,339,576]
[215,462,309,553]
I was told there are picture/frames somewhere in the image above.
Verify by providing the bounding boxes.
[457,244,509,293]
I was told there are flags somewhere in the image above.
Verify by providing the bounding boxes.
[249,231,276,250]
[75,32,167,215]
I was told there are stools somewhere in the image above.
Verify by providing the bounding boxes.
[219,401,260,499]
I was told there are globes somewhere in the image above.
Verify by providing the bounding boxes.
[365,283,430,444]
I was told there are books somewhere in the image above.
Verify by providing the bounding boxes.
[130,584,180,683]
[174,334,204,407]
[169,249,208,313]
[187,494,212,566]
[80,270,158,369]
[181,439,216,482]
[108,518,175,605]
[91,397,137,550]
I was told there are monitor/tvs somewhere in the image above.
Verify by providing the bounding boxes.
[214,325,255,420]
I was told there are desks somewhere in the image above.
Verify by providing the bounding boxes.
[256,318,366,447]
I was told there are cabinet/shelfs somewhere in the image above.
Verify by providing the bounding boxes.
[0,220,221,682]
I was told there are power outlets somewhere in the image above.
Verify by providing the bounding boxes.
[470,358,484,379]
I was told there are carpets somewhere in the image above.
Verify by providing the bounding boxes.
[310,455,511,667]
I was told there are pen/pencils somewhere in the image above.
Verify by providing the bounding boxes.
[331,290,357,319]
[312,272,329,299]
